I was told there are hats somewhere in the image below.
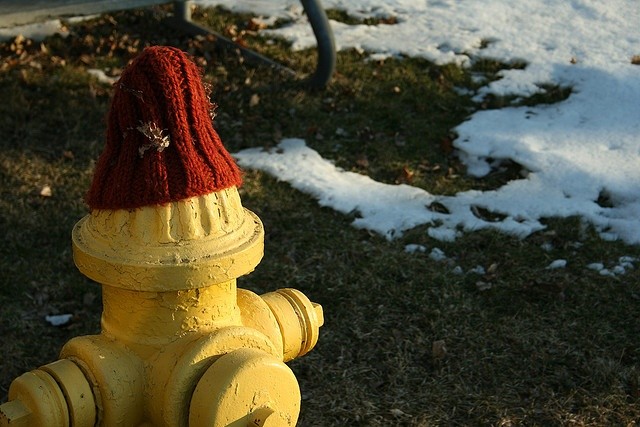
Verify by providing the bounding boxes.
[86,46,241,208]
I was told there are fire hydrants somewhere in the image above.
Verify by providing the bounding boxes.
[0,114,326,427]
